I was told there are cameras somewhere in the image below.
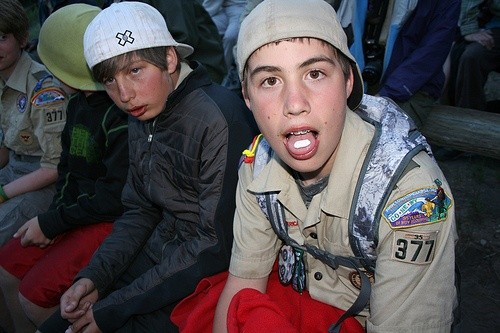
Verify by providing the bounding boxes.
[362,44,385,86]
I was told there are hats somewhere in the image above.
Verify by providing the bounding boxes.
[37,4,105,91]
[83,1,194,71]
[236,0,364,111]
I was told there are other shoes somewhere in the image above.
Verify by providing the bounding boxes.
[434,147,463,161]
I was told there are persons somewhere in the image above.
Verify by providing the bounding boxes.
[26,0,500,133]
[0,4,129,333]
[0,0,69,248]
[35,0,260,333]
[213,0,459,333]
